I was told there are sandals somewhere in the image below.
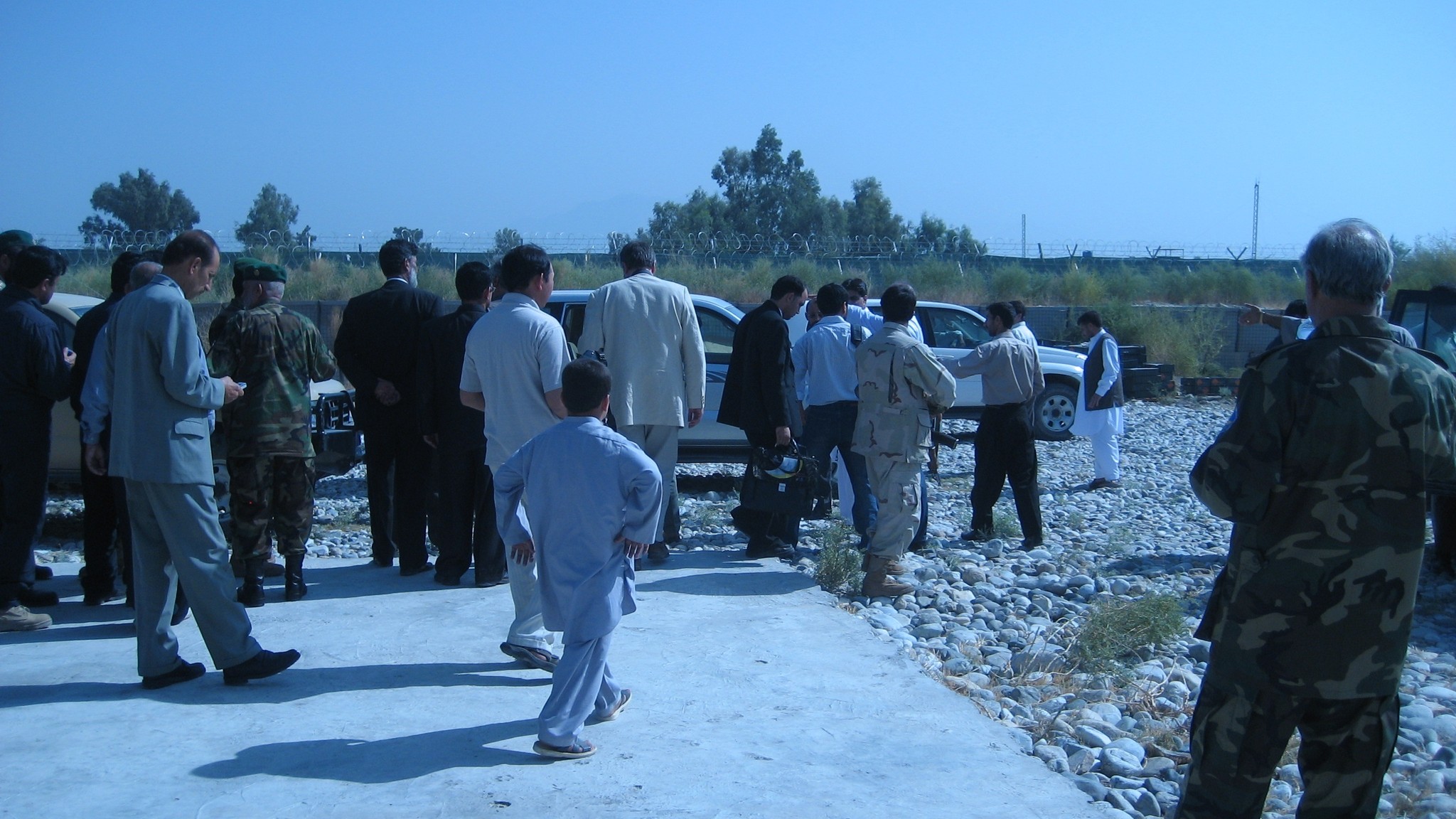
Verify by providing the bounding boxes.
[598,689,631,719]
[500,641,560,673]
[533,740,596,758]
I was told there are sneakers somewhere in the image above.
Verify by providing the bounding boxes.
[961,528,992,540]
[1022,537,1043,547]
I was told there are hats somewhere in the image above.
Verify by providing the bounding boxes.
[232,258,261,276]
[244,262,287,284]
[0,231,37,249]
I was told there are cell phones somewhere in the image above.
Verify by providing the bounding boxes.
[236,382,247,389]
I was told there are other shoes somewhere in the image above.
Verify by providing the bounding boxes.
[1090,478,1105,487]
[0,501,832,628]
[861,536,866,546]
[1099,479,1121,488]
[911,541,928,549]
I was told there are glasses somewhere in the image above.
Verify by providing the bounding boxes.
[491,287,495,291]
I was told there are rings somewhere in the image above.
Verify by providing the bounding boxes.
[233,398,235,400]
[88,466,93,469]
[1247,320,1250,323]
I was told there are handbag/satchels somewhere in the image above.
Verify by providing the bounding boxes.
[750,442,819,488]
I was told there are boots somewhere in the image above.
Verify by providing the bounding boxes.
[862,556,914,596]
[863,551,904,574]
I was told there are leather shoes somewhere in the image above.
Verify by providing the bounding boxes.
[143,659,206,690]
[223,649,300,685]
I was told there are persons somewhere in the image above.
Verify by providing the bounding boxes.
[576,238,706,561]
[0,229,191,631]
[1175,218,1456,819]
[930,315,966,348]
[206,257,336,608]
[333,238,510,587]
[80,229,300,683]
[494,357,662,759]
[459,244,571,669]
[849,285,956,596]
[715,274,929,558]
[1238,285,1456,582]
[1068,311,1124,488]
[934,300,1045,552]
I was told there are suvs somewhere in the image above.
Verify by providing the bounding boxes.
[783,297,1088,442]
[543,289,748,464]
[40,288,364,544]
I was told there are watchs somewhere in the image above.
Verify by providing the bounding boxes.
[1259,311,1266,324]
